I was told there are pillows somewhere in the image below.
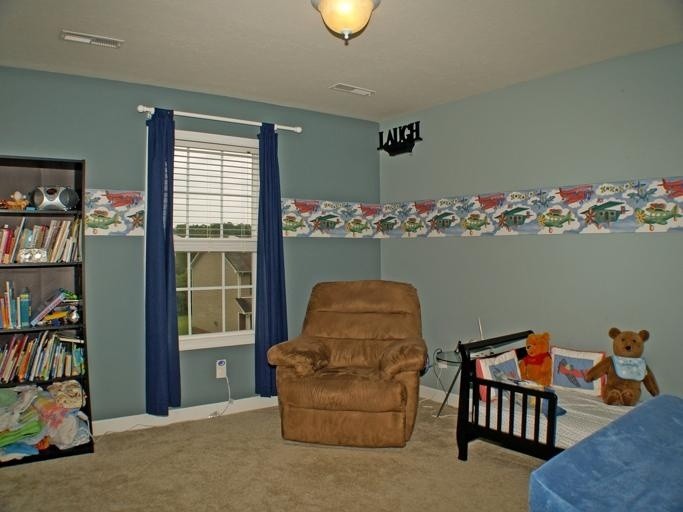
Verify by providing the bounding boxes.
[480,347,606,420]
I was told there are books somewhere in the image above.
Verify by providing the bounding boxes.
[0,216,83,264]
[0,331,86,384]
[0,279,82,329]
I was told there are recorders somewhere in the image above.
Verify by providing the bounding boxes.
[31,185,80,211]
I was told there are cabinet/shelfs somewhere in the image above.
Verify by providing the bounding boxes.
[0,153,95,467]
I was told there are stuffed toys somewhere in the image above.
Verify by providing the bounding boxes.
[518,331,553,387]
[584,326,660,407]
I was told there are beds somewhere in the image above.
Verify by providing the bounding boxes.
[453,331,635,461]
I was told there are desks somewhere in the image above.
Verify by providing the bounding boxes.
[436,351,467,419]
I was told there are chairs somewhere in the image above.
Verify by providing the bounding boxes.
[266,279,429,449]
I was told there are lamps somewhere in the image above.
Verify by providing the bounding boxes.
[311,0,381,40]
